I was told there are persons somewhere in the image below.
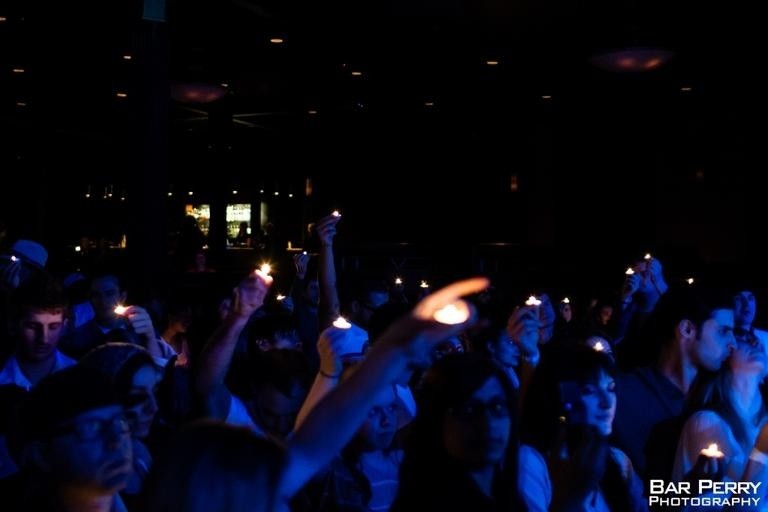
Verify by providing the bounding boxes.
[0,208,768,512]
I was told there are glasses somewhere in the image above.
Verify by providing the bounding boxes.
[75,413,131,442]
